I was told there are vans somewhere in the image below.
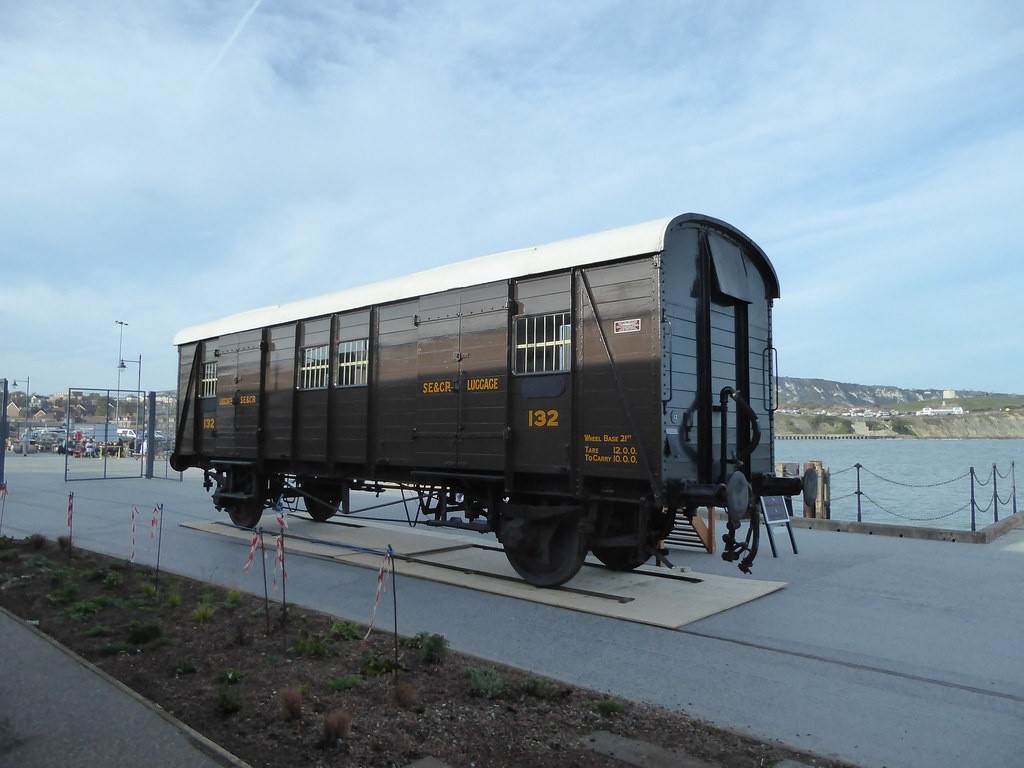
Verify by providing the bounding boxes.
[122,430,141,439]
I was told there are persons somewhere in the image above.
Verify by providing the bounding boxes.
[8,427,141,461]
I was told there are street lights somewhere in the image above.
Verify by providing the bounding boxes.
[111,320,130,417]
[10,376,31,429]
[117,353,143,451]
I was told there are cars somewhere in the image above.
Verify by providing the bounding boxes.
[145,431,167,438]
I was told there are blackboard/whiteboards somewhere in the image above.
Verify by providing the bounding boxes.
[760,495,790,524]
